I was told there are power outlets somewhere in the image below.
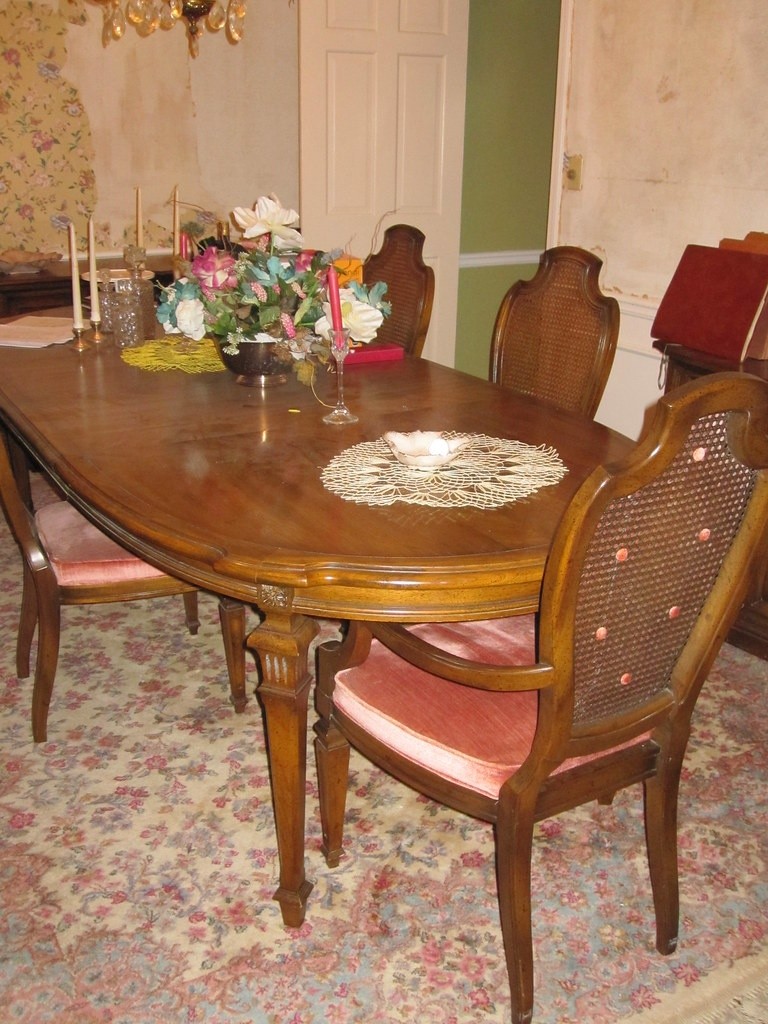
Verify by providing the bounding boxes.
[561,155,584,191]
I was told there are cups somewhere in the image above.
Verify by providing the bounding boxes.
[110,292,145,348]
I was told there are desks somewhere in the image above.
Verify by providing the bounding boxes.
[0,302,641,924]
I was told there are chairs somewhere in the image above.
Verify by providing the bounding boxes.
[314,369,768,1024]
[361,223,436,358]
[489,245,620,422]
[0,431,250,744]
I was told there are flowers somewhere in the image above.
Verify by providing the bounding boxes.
[154,191,389,386]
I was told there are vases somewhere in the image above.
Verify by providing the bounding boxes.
[208,328,297,385]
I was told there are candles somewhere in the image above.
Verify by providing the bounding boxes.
[325,264,346,349]
[69,223,85,329]
[88,211,101,321]
[180,232,187,263]
[169,183,180,281]
[134,186,144,249]
